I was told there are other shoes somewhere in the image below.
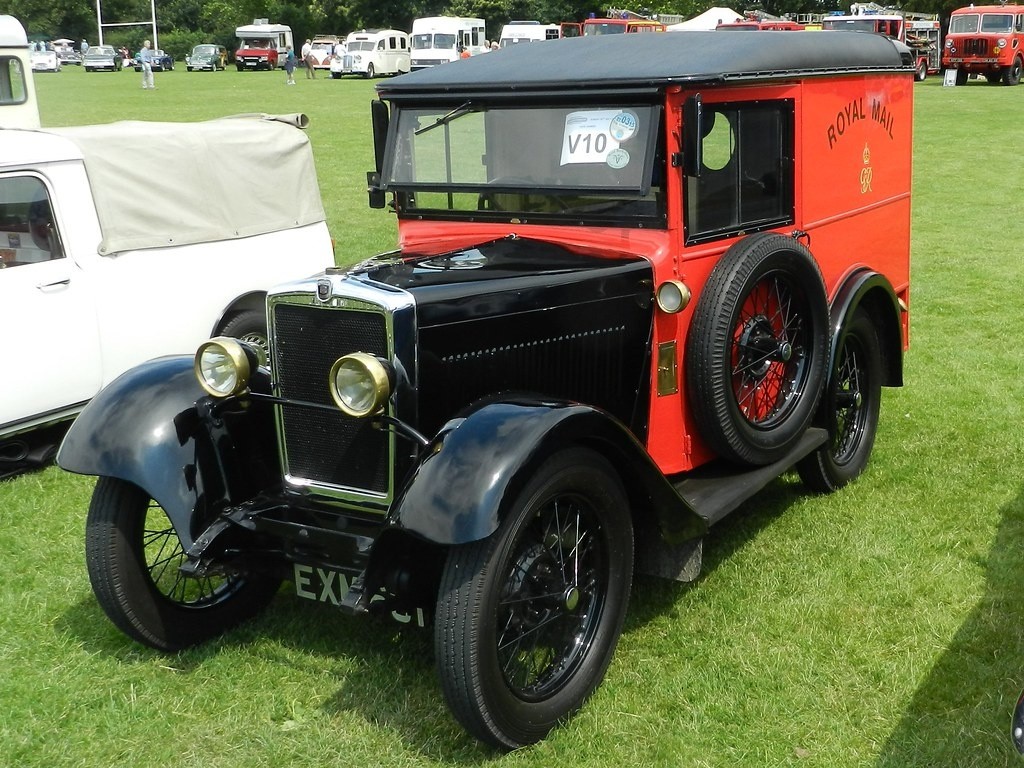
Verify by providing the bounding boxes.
[287,80,296,85]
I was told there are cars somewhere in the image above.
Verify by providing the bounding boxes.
[184,43,229,71]
[83,45,118,72]
[305,34,339,72]
[54,6,924,755]
[57,46,83,66]
[31,50,61,72]
[132,49,175,72]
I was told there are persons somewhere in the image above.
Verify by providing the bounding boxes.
[461,39,502,59]
[29,39,55,52]
[81,39,88,64]
[118,47,133,59]
[141,40,156,89]
[301,39,318,79]
[284,46,296,85]
[324,40,347,78]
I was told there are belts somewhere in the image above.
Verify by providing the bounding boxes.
[146,61,150,63]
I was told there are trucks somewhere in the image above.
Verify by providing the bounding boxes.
[942,3,1023,86]
[491,21,562,53]
[407,14,486,72]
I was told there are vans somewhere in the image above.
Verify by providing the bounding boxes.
[234,18,294,72]
[337,26,410,79]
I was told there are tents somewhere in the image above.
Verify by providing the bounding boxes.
[666,7,744,32]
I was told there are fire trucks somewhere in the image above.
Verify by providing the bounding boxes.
[560,1,941,80]
